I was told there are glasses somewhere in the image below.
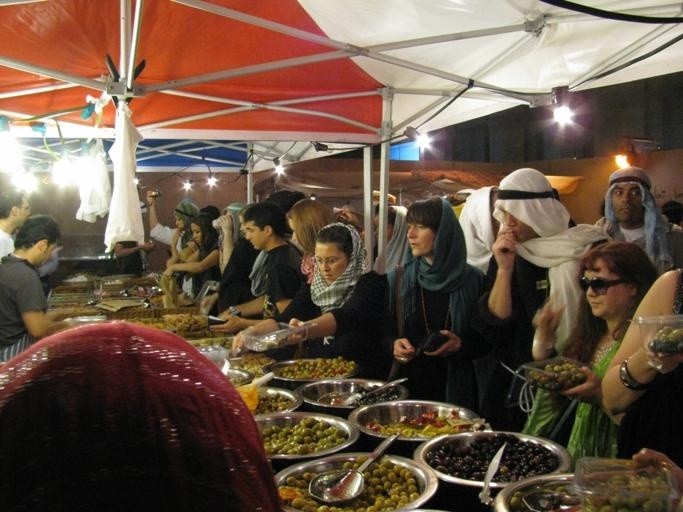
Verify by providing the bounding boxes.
[313,257,336,264]
[579,276,625,296]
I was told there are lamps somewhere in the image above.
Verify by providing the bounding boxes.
[137,33,683,199]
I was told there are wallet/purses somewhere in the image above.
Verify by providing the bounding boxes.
[422,333,448,352]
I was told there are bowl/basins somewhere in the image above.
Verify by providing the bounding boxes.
[252,386,302,422]
[348,399,482,442]
[209,316,225,331]
[263,358,361,382]
[294,379,407,408]
[253,412,359,457]
[413,431,572,488]
[274,452,438,510]
[232,357,275,367]
[223,368,252,387]
[493,473,575,511]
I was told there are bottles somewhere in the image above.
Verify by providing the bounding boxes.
[195,338,228,369]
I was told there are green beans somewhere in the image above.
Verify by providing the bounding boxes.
[254,393,295,415]
[279,454,420,511]
[263,418,346,457]
[280,355,356,380]
[529,362,586,391]
[584,475,669,510]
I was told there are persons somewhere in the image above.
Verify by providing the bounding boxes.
[0,214,73,364]
[0,318,283,510]
[0,190,31,260]
[104,164,683,512]
